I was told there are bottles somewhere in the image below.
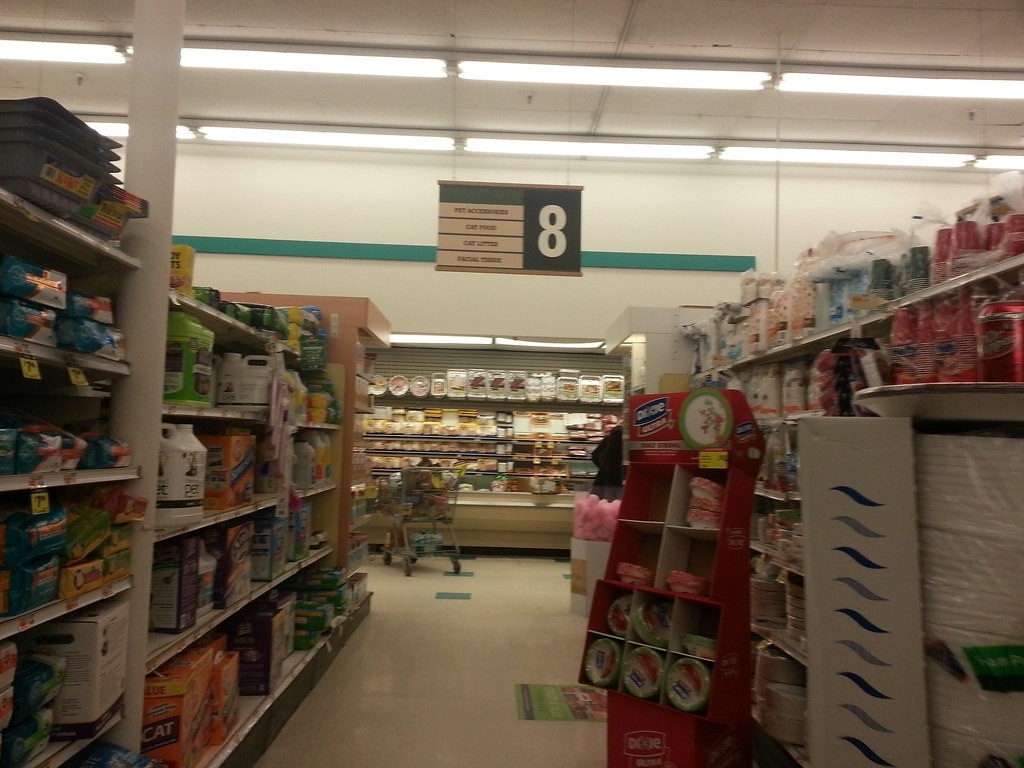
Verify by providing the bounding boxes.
[152,310,333,525]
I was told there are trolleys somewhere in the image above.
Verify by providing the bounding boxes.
[375,466,462,578]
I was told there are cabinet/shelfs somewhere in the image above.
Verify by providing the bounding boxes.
[1,184,392,768]
[701,175,1023,768]
[577,389,766,768]
[365,348,622,552]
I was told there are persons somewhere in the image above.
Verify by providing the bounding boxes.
[589,418,627,503]
